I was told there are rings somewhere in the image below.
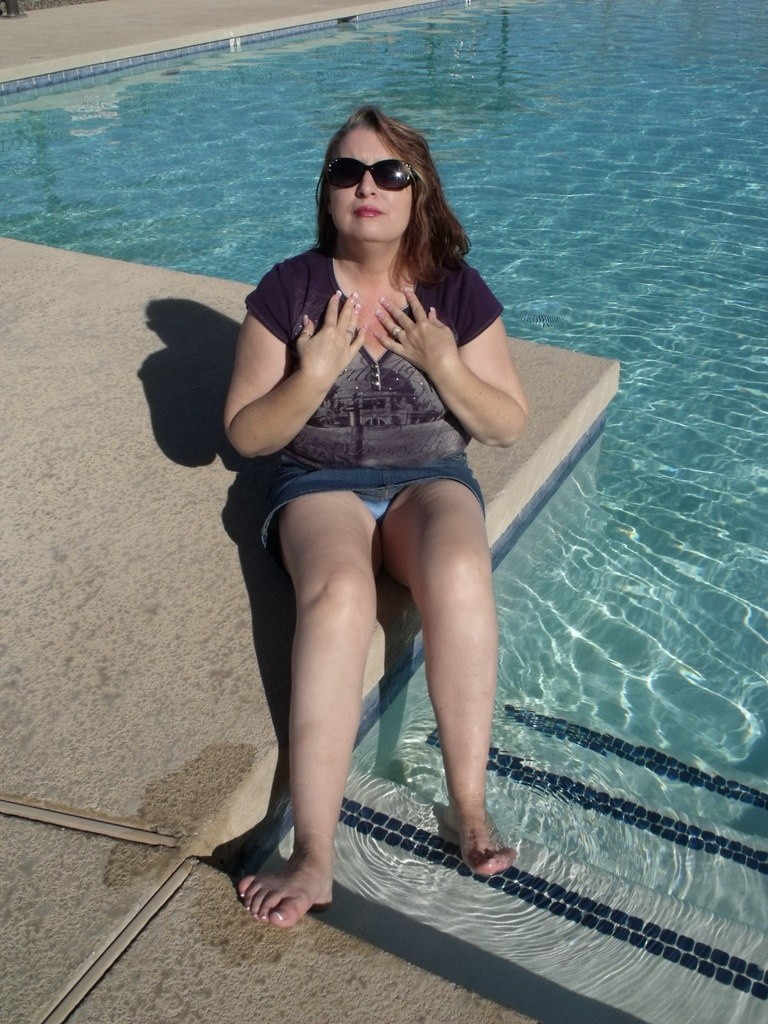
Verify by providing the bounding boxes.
[393,326,401,337]
[302,331,312,338]
[347,329,355,337]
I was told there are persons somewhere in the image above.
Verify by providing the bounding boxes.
[224,105,528,929]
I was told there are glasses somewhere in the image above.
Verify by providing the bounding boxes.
[315,158,420,193]
